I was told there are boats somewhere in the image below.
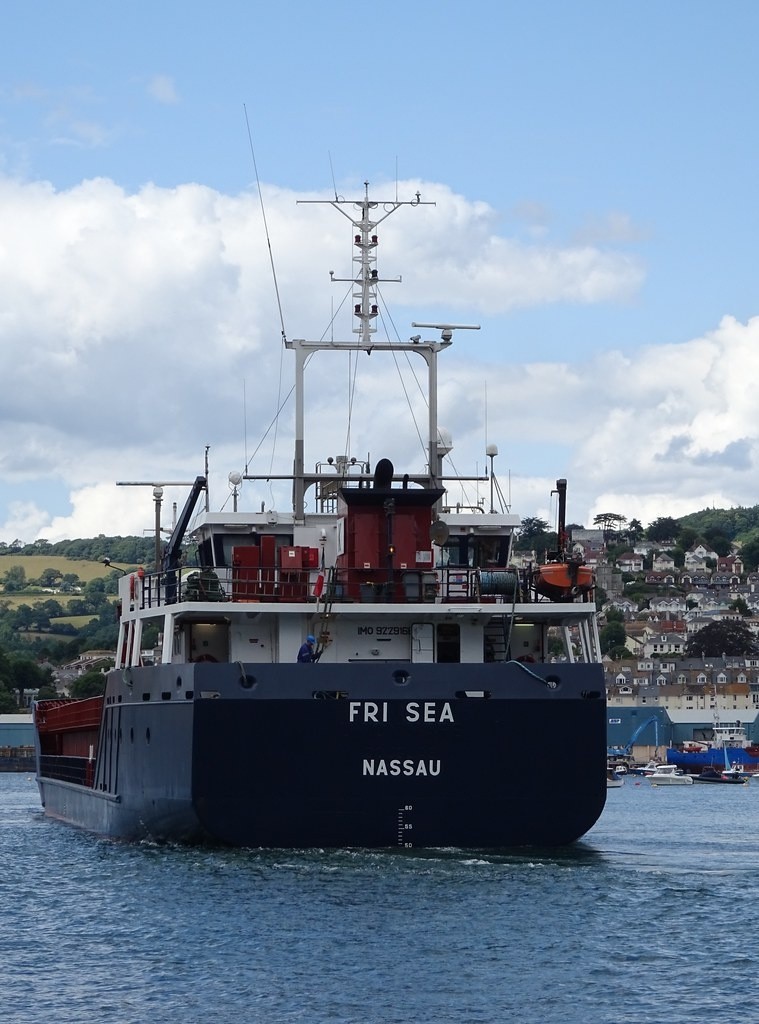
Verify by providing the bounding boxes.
[607,692,759,787]
[32,177,607,848]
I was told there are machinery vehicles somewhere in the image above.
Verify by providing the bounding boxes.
[608,714,659,763]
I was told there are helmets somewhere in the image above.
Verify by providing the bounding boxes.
[306,635,316,643]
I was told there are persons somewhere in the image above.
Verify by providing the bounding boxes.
[296,635,323,663]
